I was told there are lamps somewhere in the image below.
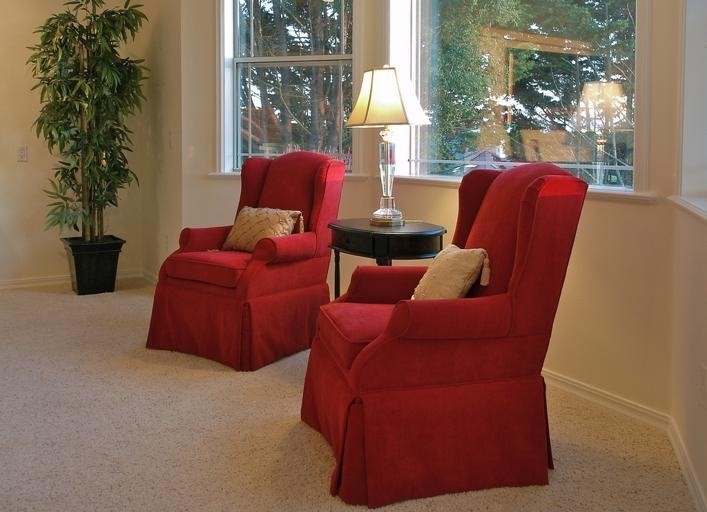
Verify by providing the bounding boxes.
[568,82,629,185]
[344,64,432,227]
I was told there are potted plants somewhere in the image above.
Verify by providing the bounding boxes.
[26,0,150,295]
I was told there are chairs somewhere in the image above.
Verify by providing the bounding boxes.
[146,151,347,372]
[300,162,589,508]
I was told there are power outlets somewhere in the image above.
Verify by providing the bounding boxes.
[16,146,27,161]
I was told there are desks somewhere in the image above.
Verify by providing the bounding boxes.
[328,219,447,301]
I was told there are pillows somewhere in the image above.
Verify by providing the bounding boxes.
[411,244,490,300]
[221,206,305,251]
